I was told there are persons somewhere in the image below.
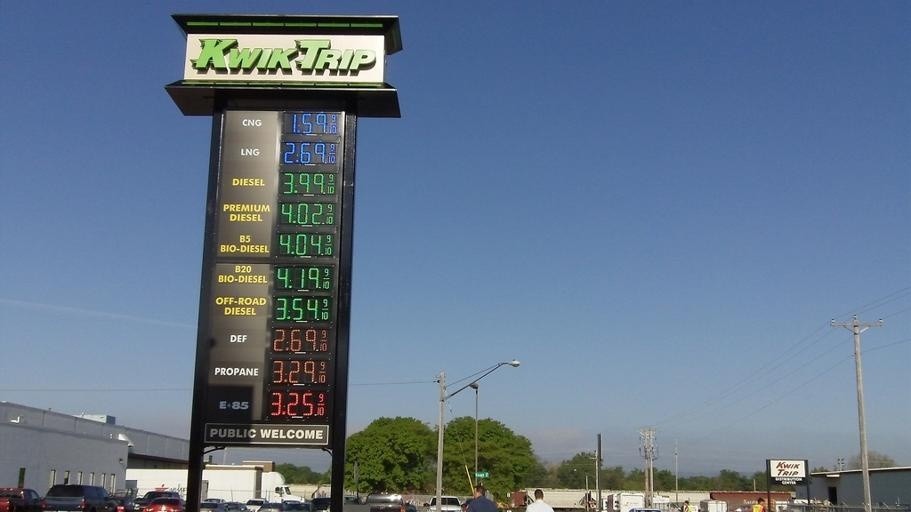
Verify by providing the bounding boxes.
[525,489,555,512]
[466,486,498,511]
[752,498,767,512]
[682,500,694,512]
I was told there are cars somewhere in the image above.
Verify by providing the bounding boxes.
[629,507,663,512]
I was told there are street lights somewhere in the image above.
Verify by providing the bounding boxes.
[572,468,590,512]
[435,358,521,512]
[468,381,480,499]
[836,457,845,471]
[673,450,679,501]
